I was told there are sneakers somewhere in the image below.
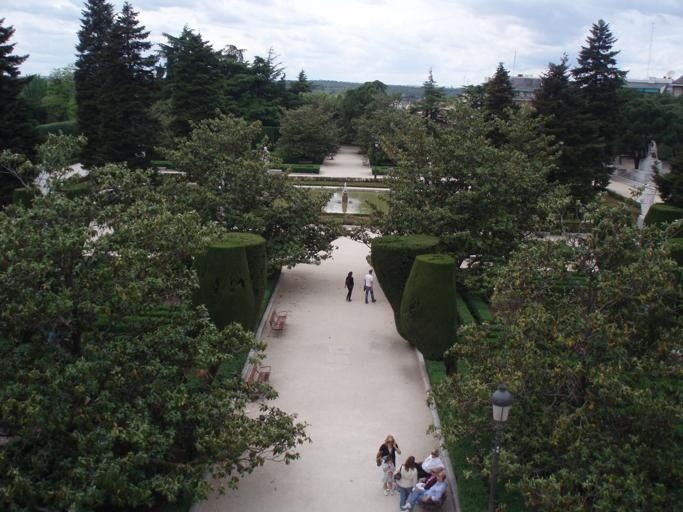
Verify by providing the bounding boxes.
[401,503,412,509]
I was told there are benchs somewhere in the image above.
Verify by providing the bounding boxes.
[241,361,271,400]
[266,308,287,338]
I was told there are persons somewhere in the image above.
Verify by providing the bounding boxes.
[363,269,377,303]
[377,435,447,512]
[345,272,354,301]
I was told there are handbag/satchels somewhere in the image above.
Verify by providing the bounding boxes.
[394,473,401,479]
[376,451,382,466]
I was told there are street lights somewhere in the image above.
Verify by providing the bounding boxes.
[488,385,513,512]
